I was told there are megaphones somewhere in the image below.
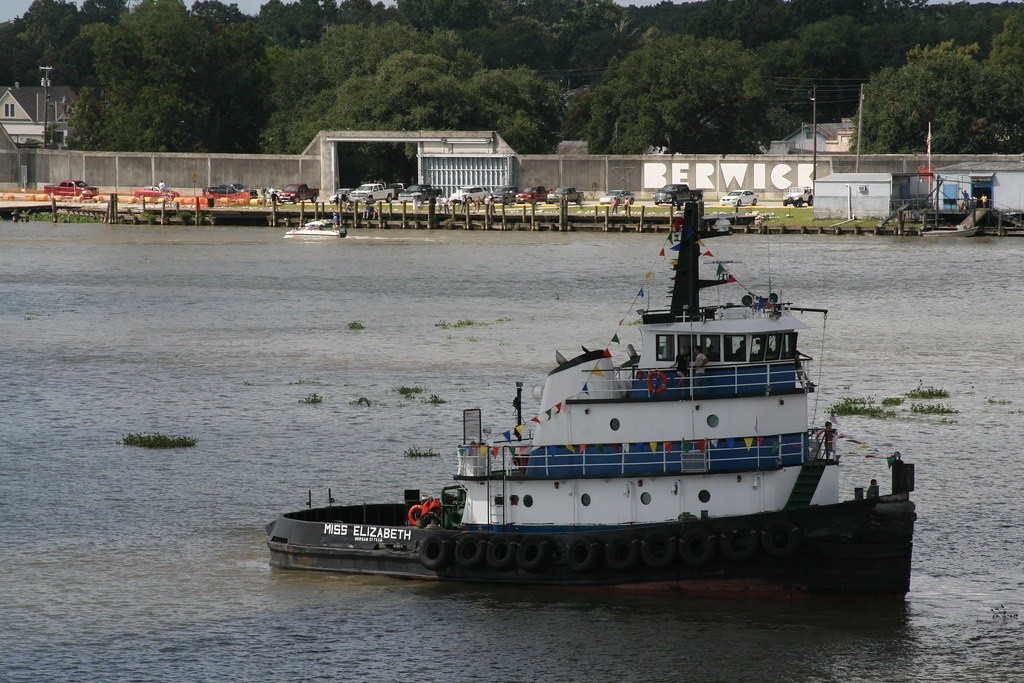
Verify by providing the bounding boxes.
[742,295,753,306]
[770,293,778,303]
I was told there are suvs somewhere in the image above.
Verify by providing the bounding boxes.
[448,186,490,204]
[783,187,813,207]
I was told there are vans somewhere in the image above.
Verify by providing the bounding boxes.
[377,181,407,200]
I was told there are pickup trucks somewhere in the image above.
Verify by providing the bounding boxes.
[547,187,584,205]
[398,185,442,203]
[208,183,258,198]
[44,180,97,198]
[516,186,552,204]
[653,184,702,206]
[278,184,319,204]
[349,184,395,204]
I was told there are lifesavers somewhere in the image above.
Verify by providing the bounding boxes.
[516,534,554,573]
[647,370,668,394]
[761,519,801,559]
[487,535,517,572]
[677,527,720,566]
[605,533,641,567]
[454,535,486,570]
[418,532,453,571]
[565,537,601,574]
[408,504,426,525]
[641,532,674,568]
[721,524,759,562]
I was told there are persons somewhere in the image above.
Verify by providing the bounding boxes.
[158,180,166,194]
[334,192,348,210]
[735,340,747,361]
[610,194,630,215]
[362,203,380,220]
[436,194,496,215]
[981,193,988,208]
[693,345,708,386]
[961,190,969,207]
[332,213,338,230]
[412,196,422,215]
[824,421,833,460]
[668,346,689,398]
[262,187,280,205]
[705,345,716,361]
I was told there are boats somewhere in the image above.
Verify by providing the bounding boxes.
[285,227,340,236]
[265,192,916,604]
[922,226,979,237]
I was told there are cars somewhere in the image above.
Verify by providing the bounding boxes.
[491,186,519,205]
[329,188,354,204]
[133,186,180,200]
[599,189,635,205]
[719,190,758,207]
